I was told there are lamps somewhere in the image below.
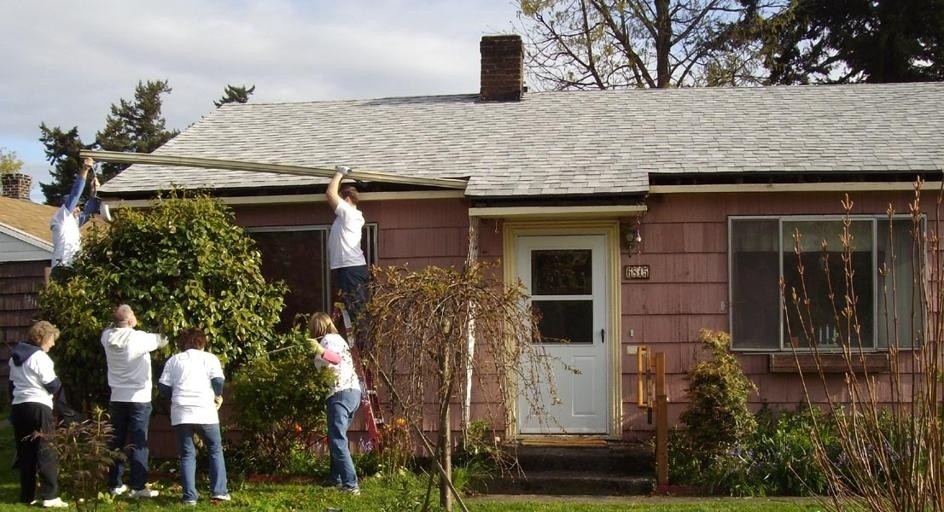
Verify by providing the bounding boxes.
[624,219,637,257]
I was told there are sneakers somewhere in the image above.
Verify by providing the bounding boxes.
[42,495,70,510]
[127,487,160,499]
[107,483,129,496]
[209,491,232,502]
[341,485,363,498]
[182,498,197,508]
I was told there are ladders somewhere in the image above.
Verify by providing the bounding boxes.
[304,302,385,458]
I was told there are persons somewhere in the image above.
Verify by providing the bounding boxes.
[307,310,362,496]
[99,304,168,498]
[47,158,99,288]
[326,165,370,351]
[156,328,232,506]
[5,321,71,508]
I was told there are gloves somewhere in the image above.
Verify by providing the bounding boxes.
[335,164,353,177]
[306,338,325,355]
